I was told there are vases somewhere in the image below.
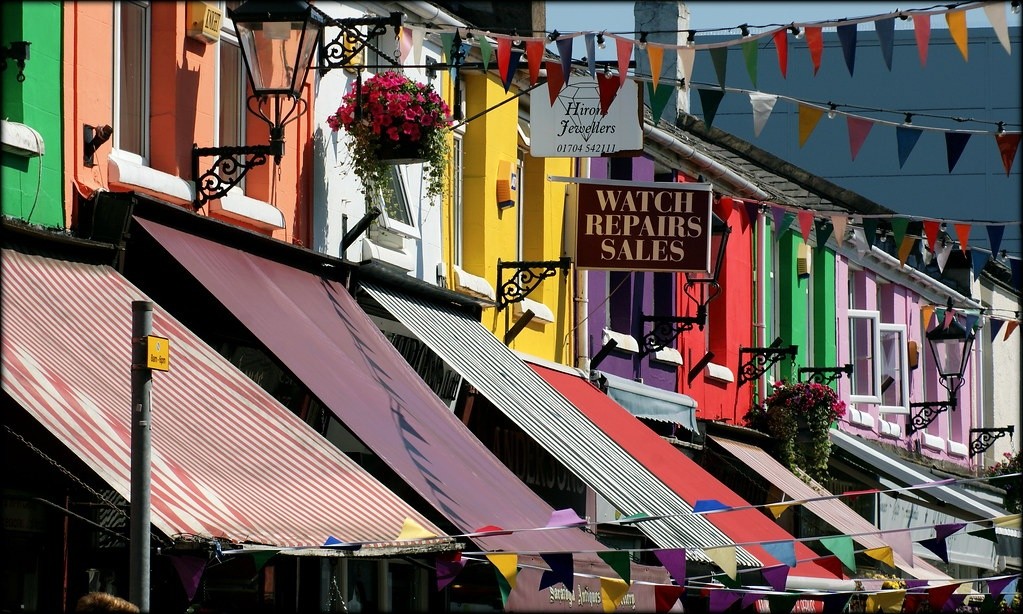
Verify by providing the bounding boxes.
[372,117,431,165]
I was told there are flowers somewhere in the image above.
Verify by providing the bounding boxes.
[741,378,847,496]
[324,68,470,237]
[975,449,1021,514]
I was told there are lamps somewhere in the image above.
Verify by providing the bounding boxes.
[633,175,732,371]
[185,0,336,210]
[905,296,976,436]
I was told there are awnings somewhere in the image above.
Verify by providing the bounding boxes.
[130,214,684,613]
[526,363,852,590]
[827,430,1022,558]
[358,282,763,573]
[0,249,464,556]
[708,434,976,596]
